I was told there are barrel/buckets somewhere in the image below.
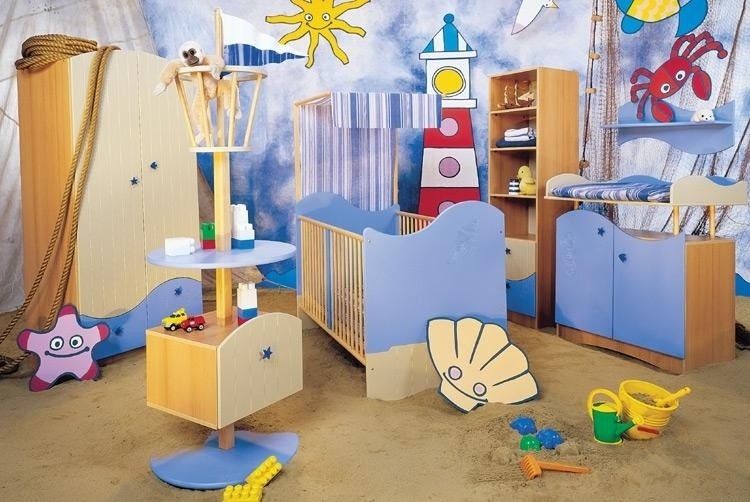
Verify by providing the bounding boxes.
[618,379,678,442]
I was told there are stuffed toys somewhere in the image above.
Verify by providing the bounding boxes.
[517,166,536,196]
[153,41,243,146]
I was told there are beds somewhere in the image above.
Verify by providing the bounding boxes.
[291,185,509,404]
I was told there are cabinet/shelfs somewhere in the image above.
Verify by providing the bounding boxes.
[143,238,305,430]
[543,169,749,376]
[15,48,207,360]
[487,65,582,332]
